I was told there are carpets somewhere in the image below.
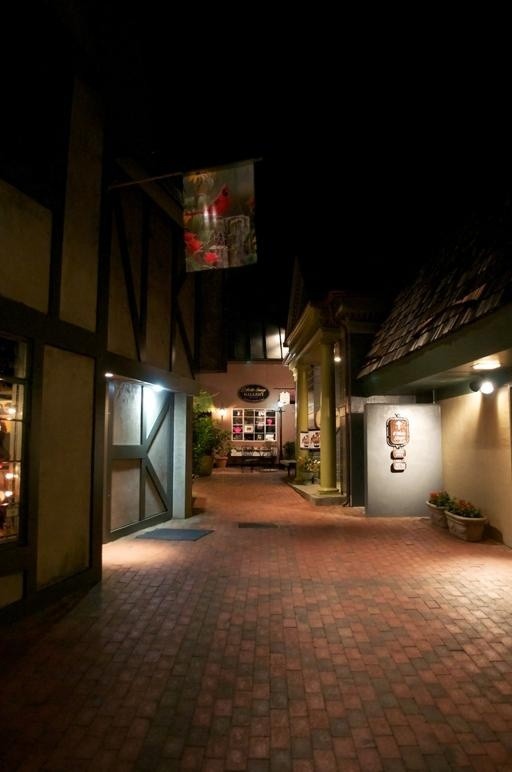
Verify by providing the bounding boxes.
[139,529,215,541]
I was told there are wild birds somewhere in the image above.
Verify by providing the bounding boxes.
[185,183,229,219]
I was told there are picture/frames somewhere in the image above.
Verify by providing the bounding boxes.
[386,417,410,446]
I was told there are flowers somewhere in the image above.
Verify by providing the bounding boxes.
[298,456,320,472]
[446,496,480,518]
[429,490,449,507]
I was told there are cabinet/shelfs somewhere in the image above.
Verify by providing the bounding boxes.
[230,447,278,467]
[230,408,282,442]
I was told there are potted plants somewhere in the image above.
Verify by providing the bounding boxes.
[192,416,233,477]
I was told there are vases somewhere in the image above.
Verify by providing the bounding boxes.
[425,500,448,528]
[302,472,314,485]
[444,510,488,542]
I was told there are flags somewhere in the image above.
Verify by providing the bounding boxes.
[177,160,259,276]
